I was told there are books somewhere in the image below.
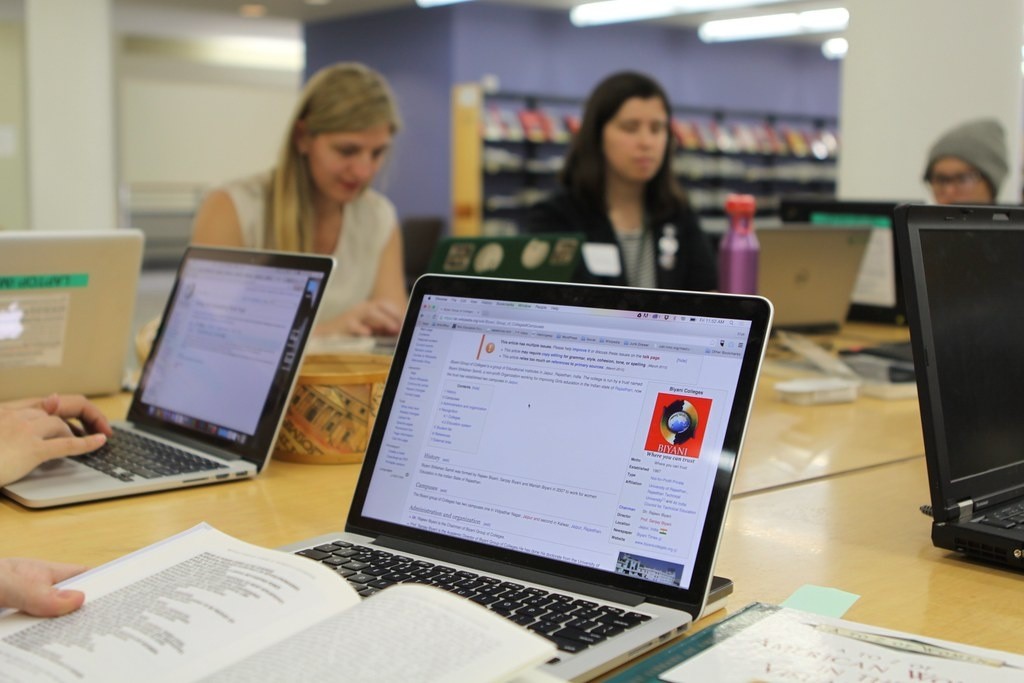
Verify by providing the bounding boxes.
[0,521,557,683]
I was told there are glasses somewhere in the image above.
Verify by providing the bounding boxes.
[931,171,971,191]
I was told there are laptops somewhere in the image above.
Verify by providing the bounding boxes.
[0,197,1023,683]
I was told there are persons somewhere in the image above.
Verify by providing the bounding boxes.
[525,72,719,294]
[0,557,91,617]
[924,117,1009,206]
[191,62,409,339]
[0,392,113,495]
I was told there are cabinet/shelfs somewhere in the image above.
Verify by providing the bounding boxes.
[449,83,840,235]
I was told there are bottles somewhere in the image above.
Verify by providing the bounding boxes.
[718,193,759,294]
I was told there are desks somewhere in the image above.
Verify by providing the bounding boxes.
[0,322,1024,683]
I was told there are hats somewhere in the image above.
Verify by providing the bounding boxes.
[923,118,1010,195]
